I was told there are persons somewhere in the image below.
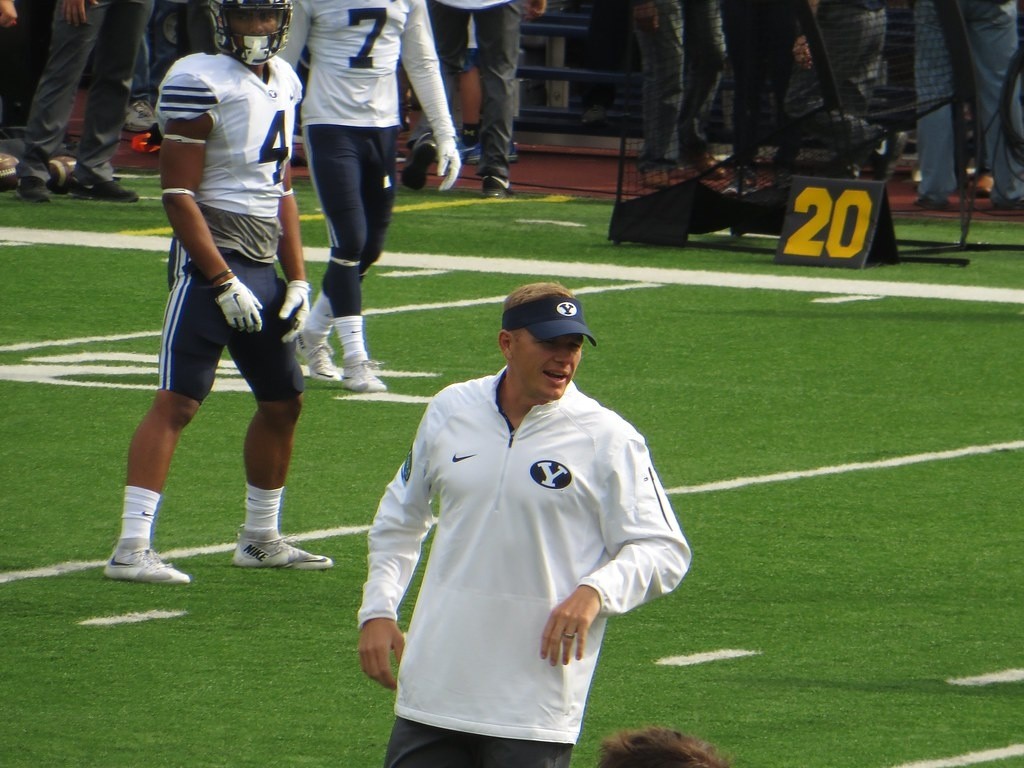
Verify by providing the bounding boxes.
[357,284,692,768]
[403,0,546,196]
[0,0,215,201]
[746,0,1024,210]
[598,727,729,768]
[279,0,461,390]
[104,0,336,583]
[635,0,729,187]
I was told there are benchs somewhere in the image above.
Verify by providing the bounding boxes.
[508,10,928,144]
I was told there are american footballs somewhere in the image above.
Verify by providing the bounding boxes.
[44,154,78,193]
[0,152,20,193]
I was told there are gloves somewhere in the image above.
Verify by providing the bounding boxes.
[279,280,311,343]
[212,275,263,333]
[437,137,461,191]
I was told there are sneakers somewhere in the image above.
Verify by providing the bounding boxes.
[401,132,437,190]
[124,99,158,132]
[292,326,341,381]
[482,175,514,198]
[342,354,387,392]
[871,129,908,183]
[232,537,332,569]
[103,548,191,584]
[16,175,50,202]
[67,173,139,203]
[458,137,517,163]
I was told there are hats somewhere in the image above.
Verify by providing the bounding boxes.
[502,296,596,346]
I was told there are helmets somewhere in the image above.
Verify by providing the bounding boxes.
[208,0,294,65]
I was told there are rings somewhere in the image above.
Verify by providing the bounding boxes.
[563,633,575,639]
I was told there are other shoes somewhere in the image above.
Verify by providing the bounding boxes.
[993,200,1024,210]
[735,162,756,188]
[643,166,701,189]
[916,193,949,210]
[684,151,727,180]
[776,168,793,193]
